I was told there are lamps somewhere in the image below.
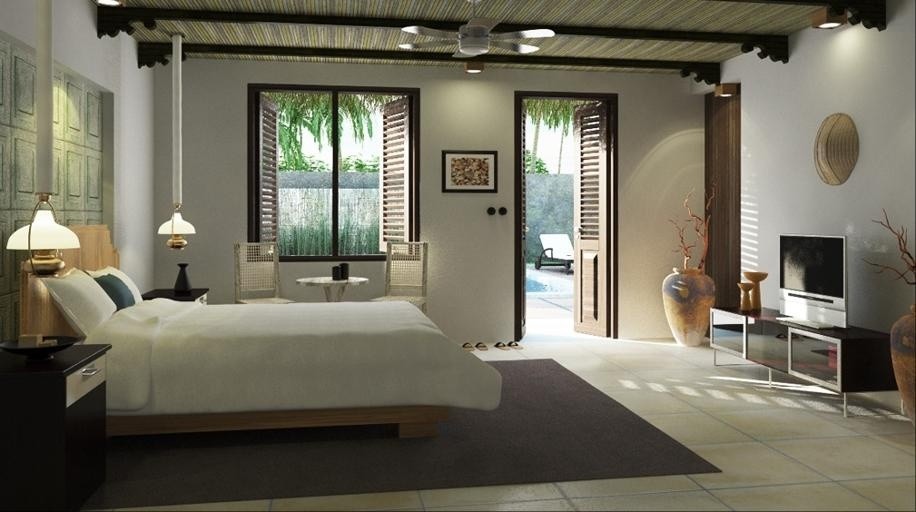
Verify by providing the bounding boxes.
[156,34,196,253]
[813,2,846,29]
[714,83,738,100]
[466,62,485,75]
[6,0,82,272]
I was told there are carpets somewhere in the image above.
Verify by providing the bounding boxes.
[1,357,726,511]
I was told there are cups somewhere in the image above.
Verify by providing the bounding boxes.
[339,263,349,280]
[332,266,341,281]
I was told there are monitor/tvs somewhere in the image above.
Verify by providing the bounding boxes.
[776,233,849,330]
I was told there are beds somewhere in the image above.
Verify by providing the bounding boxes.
[15,223,469,444]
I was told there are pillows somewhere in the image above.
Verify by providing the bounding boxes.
[96,273,136,310]
[88,266,145,304]
[37,267,117,342]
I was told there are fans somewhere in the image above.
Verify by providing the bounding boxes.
[399,0,556,60]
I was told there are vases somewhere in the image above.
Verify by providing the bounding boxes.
[173,262,192,296]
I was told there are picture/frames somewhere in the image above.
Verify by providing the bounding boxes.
[441,149,500,193]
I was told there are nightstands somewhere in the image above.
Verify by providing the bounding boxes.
[142,288,210,306]
[0,344,116,509]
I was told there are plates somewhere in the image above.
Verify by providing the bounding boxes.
[0,337,74,360]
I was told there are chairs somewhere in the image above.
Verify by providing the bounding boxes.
[371,238,432,317]
[536,234,574,274]
[232,239,296,302]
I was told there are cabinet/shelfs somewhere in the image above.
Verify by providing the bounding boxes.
[707,305,901,419]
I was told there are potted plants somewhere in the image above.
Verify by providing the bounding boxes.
[858,209,914,426]
[658,183,718,350]
